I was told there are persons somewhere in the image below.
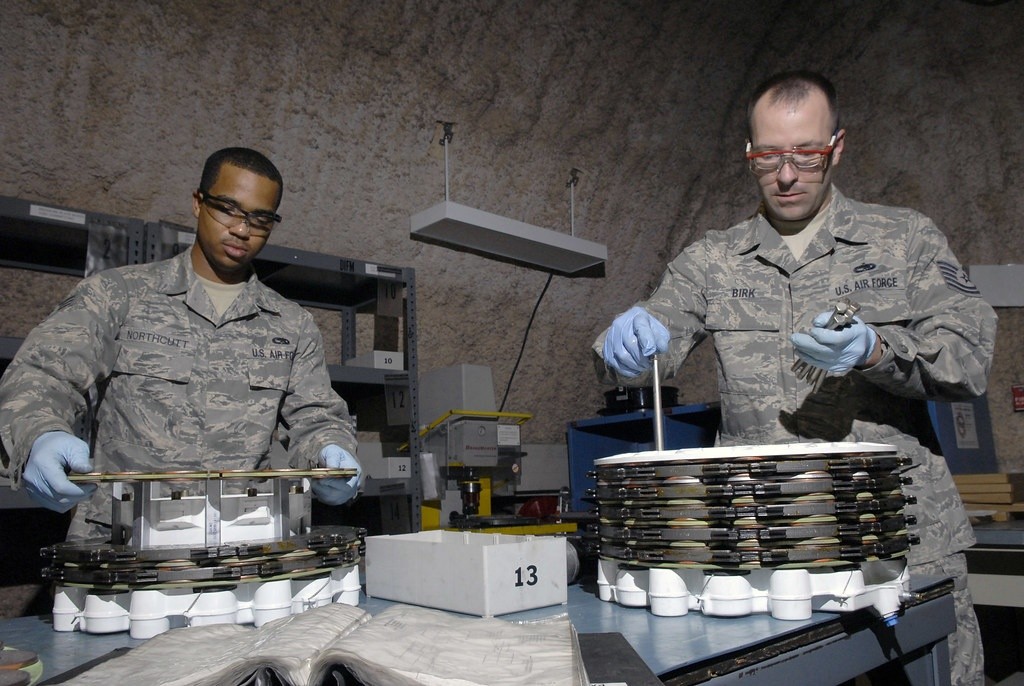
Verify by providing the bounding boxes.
[592,71,1000,686]
[0,148,362,544]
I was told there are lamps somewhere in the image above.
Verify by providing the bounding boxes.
[411,125,608,275]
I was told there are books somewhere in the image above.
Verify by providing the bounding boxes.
[51,604,591,686]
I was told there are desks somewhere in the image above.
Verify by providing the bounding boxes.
[0,574,959,686]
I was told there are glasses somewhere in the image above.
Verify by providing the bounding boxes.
[197,188,282,232]
[745,128,840,178]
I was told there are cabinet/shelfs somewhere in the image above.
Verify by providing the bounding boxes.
[0,194,423,534]
[568,381,998,515]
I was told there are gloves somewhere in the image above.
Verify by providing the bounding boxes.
[603,306,670,378]
[310,443,362,505]
[19,432,98,513]
[790,312,877,377]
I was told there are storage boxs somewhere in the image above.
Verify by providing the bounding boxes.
[365,529,568,617]
